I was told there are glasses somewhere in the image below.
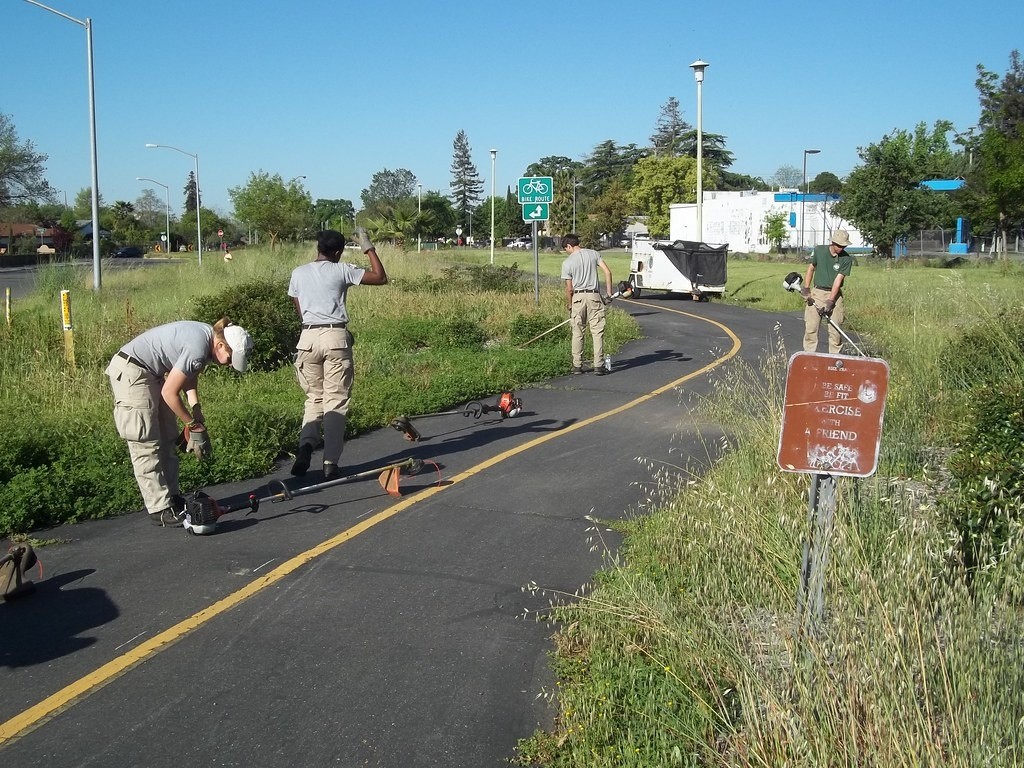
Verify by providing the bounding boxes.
[226,352,232,364]
[834,243,847,248]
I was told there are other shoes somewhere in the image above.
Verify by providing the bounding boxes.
[573,367,583,374]
[291,442,313,479]
[322,462,351,482]
[593,366,610,375]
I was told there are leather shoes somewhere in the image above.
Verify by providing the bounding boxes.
[150,495,187,529]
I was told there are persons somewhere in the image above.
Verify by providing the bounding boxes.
[560,234,613,375]
[153,243,233,263]
[104,317,252,527]
[804,229,852,354]
[458,238,482,248]
[287,226,389,480]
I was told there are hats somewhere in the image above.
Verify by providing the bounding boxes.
[223,325,253,373]
[828,229,853,246]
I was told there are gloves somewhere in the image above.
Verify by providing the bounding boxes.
[825,299,836,318]
[603,294,612,305]
[174,424,214,463]
[350,226,376,254]
[800,286,811,304]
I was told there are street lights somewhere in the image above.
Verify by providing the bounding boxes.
[417,184,423,253]
[689,58,711,241]
[802,149,822,251]
[489,149,499,265]
[136,178,170,254]
[145,143,203,267]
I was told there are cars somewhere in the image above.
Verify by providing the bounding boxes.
[110,246,144,258]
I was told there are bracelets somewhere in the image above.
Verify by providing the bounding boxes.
[193,402,201,411]
[186,419,198,429]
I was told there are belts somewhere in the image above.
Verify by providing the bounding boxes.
[302,324,346,329]
[116,349,150,372]
[814,285,832,291]
[574,290,599,293]
